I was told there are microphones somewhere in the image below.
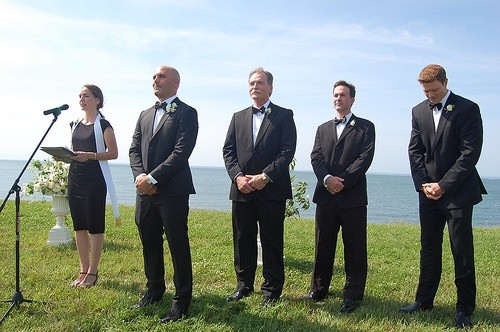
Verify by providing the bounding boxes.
[43,104,69,115]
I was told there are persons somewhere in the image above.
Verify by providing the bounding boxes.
[129,66,199,323]
[222,68,297,305]
[399,65,488,329]
[51,84,118,287]
[308,81,375,313]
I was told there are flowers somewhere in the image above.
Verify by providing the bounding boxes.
[23,157,67,194]
[264,108,274,115]
[349,120,357,127]
[165,103,177,111]
[445,105,455,112]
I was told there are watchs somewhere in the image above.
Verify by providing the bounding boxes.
[261,173,269,184]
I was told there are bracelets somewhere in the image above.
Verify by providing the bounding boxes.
[95,153,96,160]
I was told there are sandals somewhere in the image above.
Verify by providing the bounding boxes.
[76,270,99,289]
[69,272,88,288]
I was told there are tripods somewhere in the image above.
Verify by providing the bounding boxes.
[0,111,61,324]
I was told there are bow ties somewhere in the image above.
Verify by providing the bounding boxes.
[334,116,347,125]
[429,102,442,111]
[252,106,265,114]
[155,101,168,112]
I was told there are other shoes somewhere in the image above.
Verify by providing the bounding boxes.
[225,291,252,303]
[339,301,359,314]
[258,297,277,309]
[160,305,189,323]
[300,291,327,304]
[127,298,162,312]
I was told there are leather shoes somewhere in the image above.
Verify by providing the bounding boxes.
[398,301,433,315]
[455,311,472,330]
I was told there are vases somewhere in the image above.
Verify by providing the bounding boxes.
[47,196,72,241]
